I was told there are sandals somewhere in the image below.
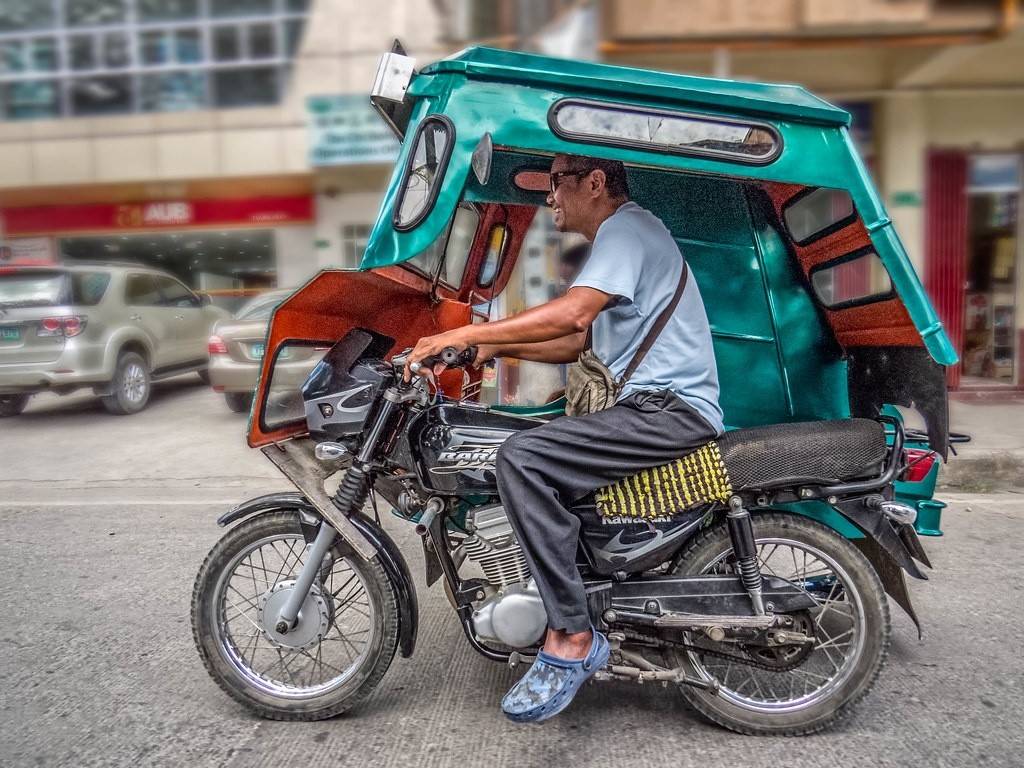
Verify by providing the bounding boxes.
[500,625,610,722]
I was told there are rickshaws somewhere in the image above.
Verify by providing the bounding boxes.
[188,41,972,740]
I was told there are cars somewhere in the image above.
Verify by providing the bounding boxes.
[207,287,328,414]
[0,257,232,419]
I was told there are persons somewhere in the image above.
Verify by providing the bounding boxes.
[404,153,726,722]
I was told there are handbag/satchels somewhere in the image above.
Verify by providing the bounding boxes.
[565,348,618,416]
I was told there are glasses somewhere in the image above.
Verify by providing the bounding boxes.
[550,170,591,192]
